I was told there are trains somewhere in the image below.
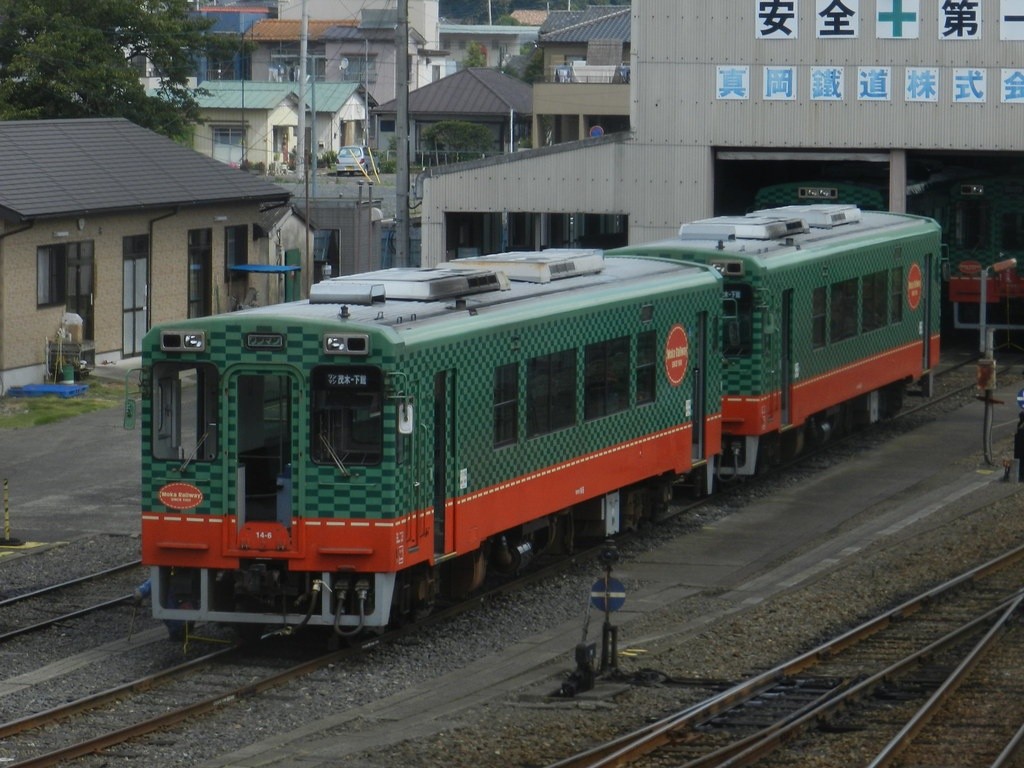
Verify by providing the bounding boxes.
[124,204,942,648]
[754,174,1024,330]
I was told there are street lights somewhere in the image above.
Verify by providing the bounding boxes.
[980,256,1017,354]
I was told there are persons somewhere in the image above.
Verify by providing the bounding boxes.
[292,146,297,170]
[1017,412,1024,483]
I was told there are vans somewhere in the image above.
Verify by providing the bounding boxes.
[336,145,380,176]
